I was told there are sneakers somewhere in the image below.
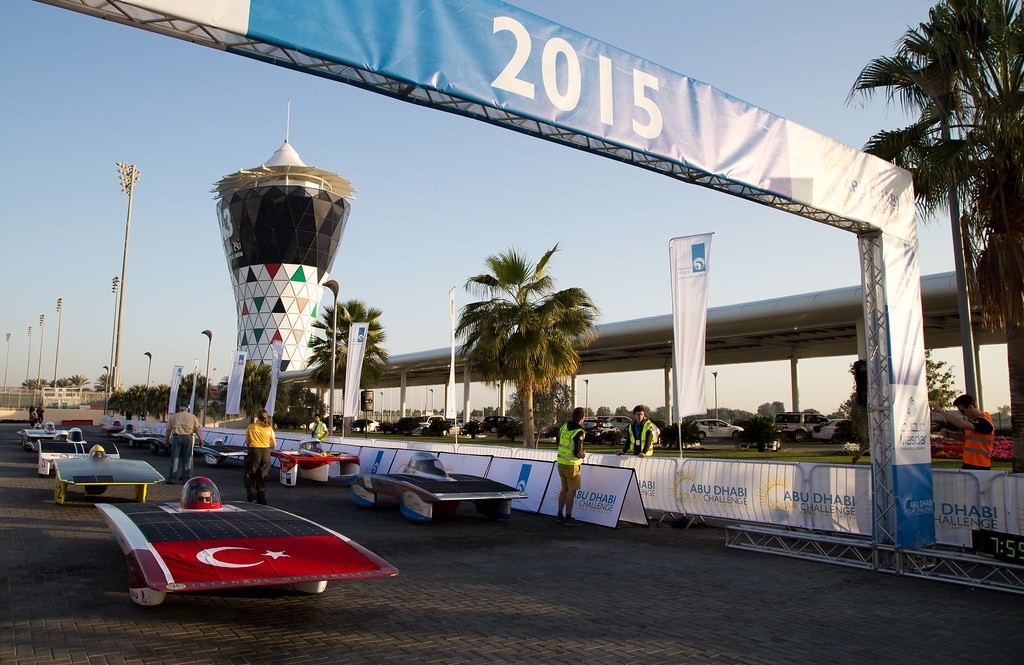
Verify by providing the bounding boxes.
[564,517,583,526]
[556,513,565,523]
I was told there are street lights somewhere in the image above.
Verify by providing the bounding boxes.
[380,391,384,423]
[37,314,46,389]
[53,296,64,388]
[583,378,589,417]
[4,333,12,393]
[142,352,152,420]
[713,371,719,418]
[102,365,109,415]
[26,327,32,389]
[200,328,213,431]
[429,388,435,414]
[497,384,500,416]
[111,159,141,393]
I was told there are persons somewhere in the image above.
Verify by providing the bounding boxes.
[554,407,586,525]
[94,452,101,457]
[36,404,44,423]
[196,488,210,503]
[126,408,131,420]
[29,406,36,427]
[929,394,994,470]
[312,414,329,441]
[244,410,277,505]
[617,406,655,458]
[165,402,204,485]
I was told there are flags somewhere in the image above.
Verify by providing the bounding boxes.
[264,340,283,416]
[446,289,457,419]
[190,366,197,413]
[226,351,247,414]
[670,233,712,418]
[168,365,184,413]
[344,323,369,417]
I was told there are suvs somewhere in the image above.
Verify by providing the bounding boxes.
[770,411,829,443]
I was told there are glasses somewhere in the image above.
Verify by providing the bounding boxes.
[198,496,210,502]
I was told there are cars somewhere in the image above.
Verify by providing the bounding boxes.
[323,414,342,430]
[544,414,632,445]
[811,419,853,444]
[690,418,745,441]
[350,418,381,434]
[386,414,524,442]
